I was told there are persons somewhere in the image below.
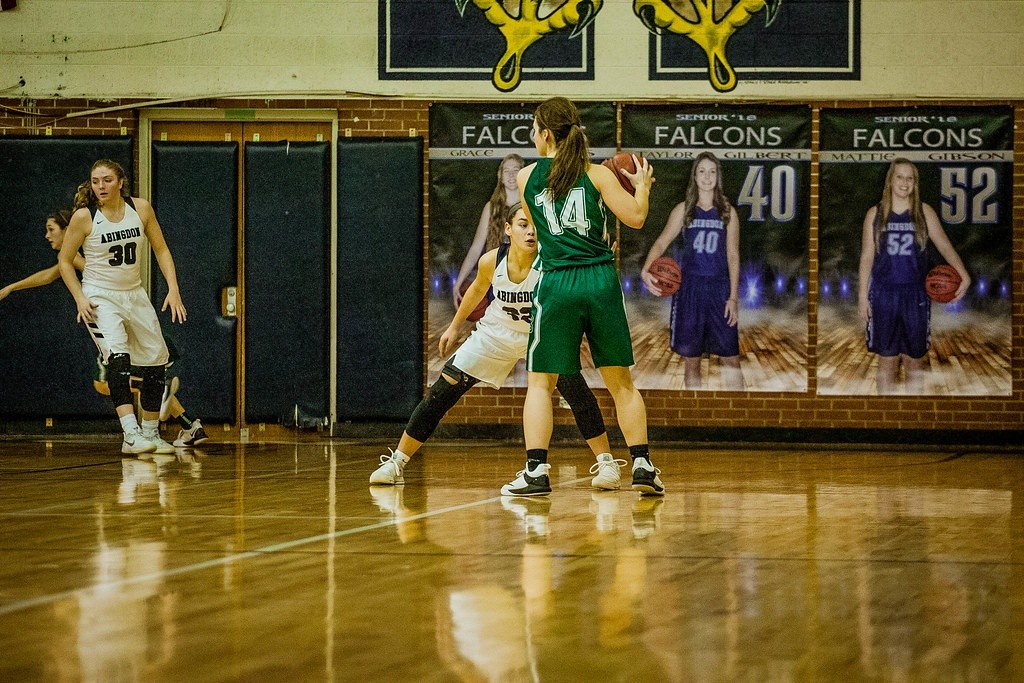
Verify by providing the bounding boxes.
[640,150,744,391]
[367,200,624,492]
[453,154,525,313]
[858,158,971,395]
[499,97,669,497]
[0,207,210,447]
[57,159,187,455]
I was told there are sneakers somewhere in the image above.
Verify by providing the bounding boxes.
[631,457,664,495]
[143,430,175,453]
[121,432,157,454]
[591,460,620,490]
[370,463,407,485]
[173,419,208,446]
[500,461,552,496]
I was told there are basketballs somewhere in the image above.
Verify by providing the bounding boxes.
[647,257,683,297]
[602,153,652,199]
[925,265,962,303]
[457,282,489,323]
